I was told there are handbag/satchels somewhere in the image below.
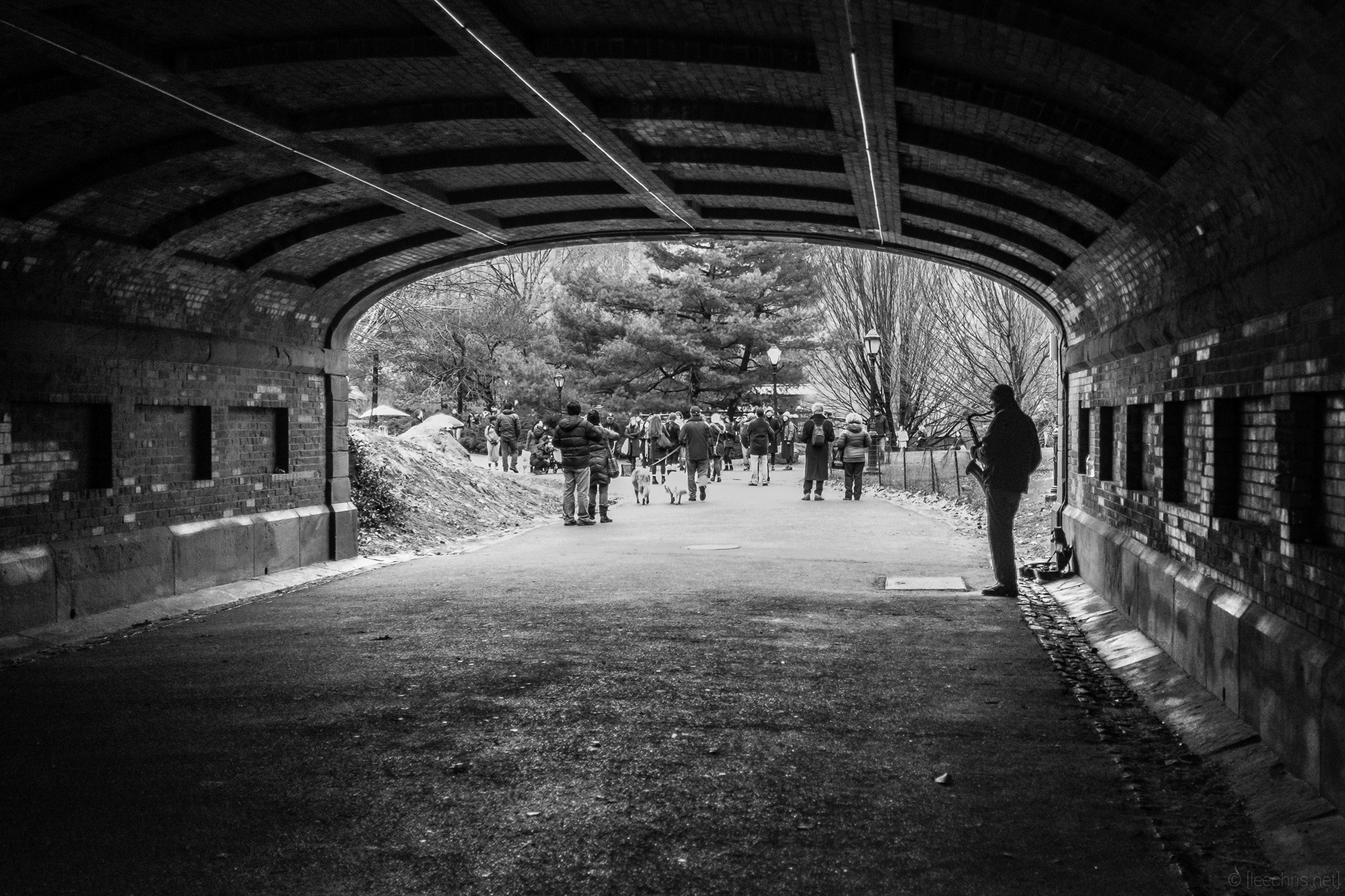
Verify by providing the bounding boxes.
[713,446,717,456]
[621,437,631,455]
[656,432,673,449]
[604,428,619,478]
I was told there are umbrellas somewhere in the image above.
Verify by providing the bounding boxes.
[423,413,465,432]
[358,404,410,435]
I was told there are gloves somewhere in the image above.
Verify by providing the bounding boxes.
[490,440,498,446]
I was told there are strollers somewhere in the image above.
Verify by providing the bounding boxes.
[545,436,564,475]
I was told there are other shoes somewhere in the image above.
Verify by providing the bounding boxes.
[843,497,852,500]
[488,461,543,475]
[749,483,757,486]
[982,581,1018,597]
[814,494,824,500]
[564,519,577,526]
[783,465,792,470]
[801,493,810,500]
[577,519,597,525]
[771,466,775,470]
[855,496,860,500]
[651,465,749,484]
[689,497,696,501]
[767,477,770,481]
[763,483,768,486]
[699,485,706,501]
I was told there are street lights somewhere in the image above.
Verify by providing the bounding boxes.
[766,344,783,445]
[553,371,565,420]
[862,328,884,475]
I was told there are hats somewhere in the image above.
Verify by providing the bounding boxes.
[990,384,1015,404]
[587,410,600,424]
[689,406,700,416]
[606,412,614,417]
[782,411,791,418]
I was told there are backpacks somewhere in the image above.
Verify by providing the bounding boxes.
[809,419,827,445]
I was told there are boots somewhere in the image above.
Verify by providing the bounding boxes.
[588,505,596,519]
[599,505,613,522]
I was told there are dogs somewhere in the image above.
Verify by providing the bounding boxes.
[664,471,688,504]
[632,469,650,505]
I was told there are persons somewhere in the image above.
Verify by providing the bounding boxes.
[466,401,521,473]
[824,410,838,469]
[873,406,890,463]
[896,426,909,452]
[451,408,464,441]
[970,384,1042,596]
[552,400,602,525]
[949,430,967,451]
[625,405,794,501]
[411,409,423,426]
[601,412,621,454]
[800,403,835,500]
[525,420,553,474]
[917,425,928,447]
[837,413,872,500]
[585,409,619,521]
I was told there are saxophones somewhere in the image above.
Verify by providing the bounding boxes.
[965,409,995,495]
